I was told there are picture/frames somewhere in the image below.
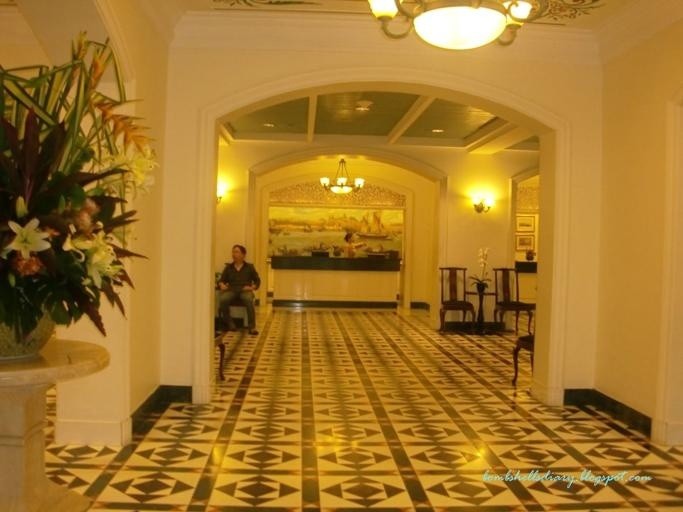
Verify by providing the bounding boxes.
[515,215,535,232]
[515,235,536,251]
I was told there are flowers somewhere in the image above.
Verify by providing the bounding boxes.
[0,31,154,336]
[470,248,492,286]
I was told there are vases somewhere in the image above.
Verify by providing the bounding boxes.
[0,289,56,361]
[477,285,485,334]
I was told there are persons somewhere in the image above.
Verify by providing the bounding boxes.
[333,232,367,257]
[309,243,332,257]
[219,244,261,334]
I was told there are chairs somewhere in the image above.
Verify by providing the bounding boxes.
[438,266,476,334]
[213,327,228,381]
[512,333,535,384]
[216,263,259,331]
[492,268,536,335]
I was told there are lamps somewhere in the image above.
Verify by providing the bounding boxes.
[471,187,493,213]
[320,159,365,194]
[368,0,601,50]
[216,182,230,204]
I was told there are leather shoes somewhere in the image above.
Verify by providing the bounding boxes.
[249,328,258,334]
